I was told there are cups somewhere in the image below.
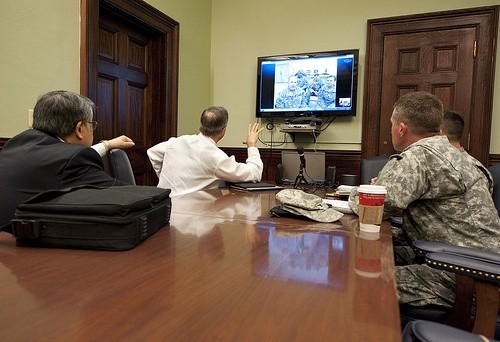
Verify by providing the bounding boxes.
[357,184,388,234]
[355,232,382,278]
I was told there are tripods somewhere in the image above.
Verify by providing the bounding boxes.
[294,162,309,189]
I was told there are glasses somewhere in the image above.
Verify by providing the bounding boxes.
[314,74,319,77]
[87,121,98,130]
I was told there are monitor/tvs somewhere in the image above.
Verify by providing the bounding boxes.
[255,49,359,118]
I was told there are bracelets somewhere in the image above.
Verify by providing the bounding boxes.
[103,140,110,151]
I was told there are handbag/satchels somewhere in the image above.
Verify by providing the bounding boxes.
[10,185,172,252]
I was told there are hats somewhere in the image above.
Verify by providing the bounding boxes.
[275,189,345,222]
[313,70,319,75]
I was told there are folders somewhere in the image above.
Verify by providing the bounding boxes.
[229,182,285,191]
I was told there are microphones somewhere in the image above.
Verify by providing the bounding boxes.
[297,145,306,169]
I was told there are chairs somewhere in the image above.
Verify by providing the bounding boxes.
[108,149,135,185]
[363,157,500,342]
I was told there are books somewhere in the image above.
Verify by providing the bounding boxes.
[323,199,354,214]
[231,183,284,191]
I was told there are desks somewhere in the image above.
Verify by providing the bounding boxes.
[0,183,402,342]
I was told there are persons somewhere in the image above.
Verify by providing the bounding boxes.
[348,93,500,308]
[275,70,336,109]
[0,90,135,234]
[147,106,265,197]
